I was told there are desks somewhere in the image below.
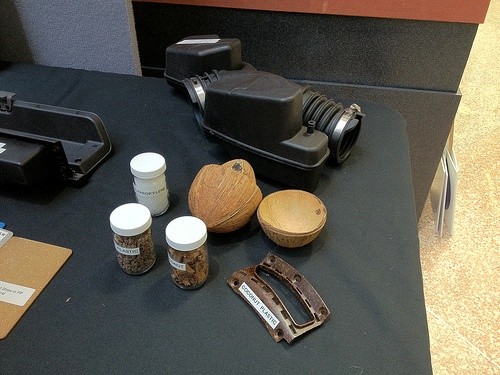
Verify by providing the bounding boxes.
[1,59,432,375]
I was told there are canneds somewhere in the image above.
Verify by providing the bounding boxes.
[130,152,169,217]
[109,203,157,275]
[164,215,210,290]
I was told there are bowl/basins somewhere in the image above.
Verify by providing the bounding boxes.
[257,189,328,248]
